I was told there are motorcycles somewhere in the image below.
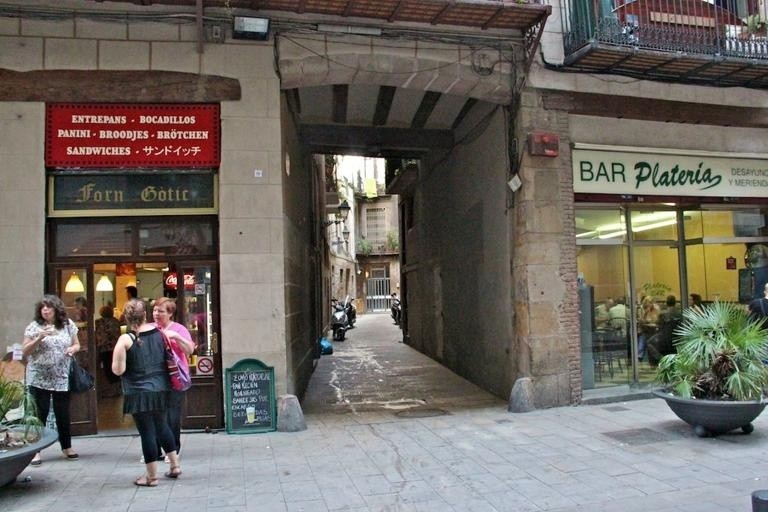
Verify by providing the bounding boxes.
[389,293,401,325]
[331,297,356,341]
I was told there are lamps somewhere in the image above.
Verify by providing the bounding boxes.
[94,272,113,292]
[64,272,84,292]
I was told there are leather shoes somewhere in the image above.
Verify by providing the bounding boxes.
[63,448,79,459]
[31,458,42,466]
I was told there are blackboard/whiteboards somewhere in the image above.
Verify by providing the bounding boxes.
[230,370,271,429]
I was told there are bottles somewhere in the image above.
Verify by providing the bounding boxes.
[187,297,197,310]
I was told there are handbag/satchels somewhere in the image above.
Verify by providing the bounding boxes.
[148,323,192,391]
[69,362,94,392]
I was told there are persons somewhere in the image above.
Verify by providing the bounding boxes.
[119,286,144,323]
[22,294,82,466]
[141,296,196,464]
[595,287,709,366]
[96,306,121,397]
[72,296,88,322]
[748,282,768,331]
[111,299,183,487]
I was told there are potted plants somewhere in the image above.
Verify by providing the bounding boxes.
[651,295,767,436]
[0,366,59,485]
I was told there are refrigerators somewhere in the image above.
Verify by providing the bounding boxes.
[162,270,208,372]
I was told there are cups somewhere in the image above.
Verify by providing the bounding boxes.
[47,320,56,335]
[187,353,197,364]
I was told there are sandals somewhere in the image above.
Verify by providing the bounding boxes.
[134,452,181,486]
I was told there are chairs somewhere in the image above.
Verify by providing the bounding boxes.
[594,305,682,381]
